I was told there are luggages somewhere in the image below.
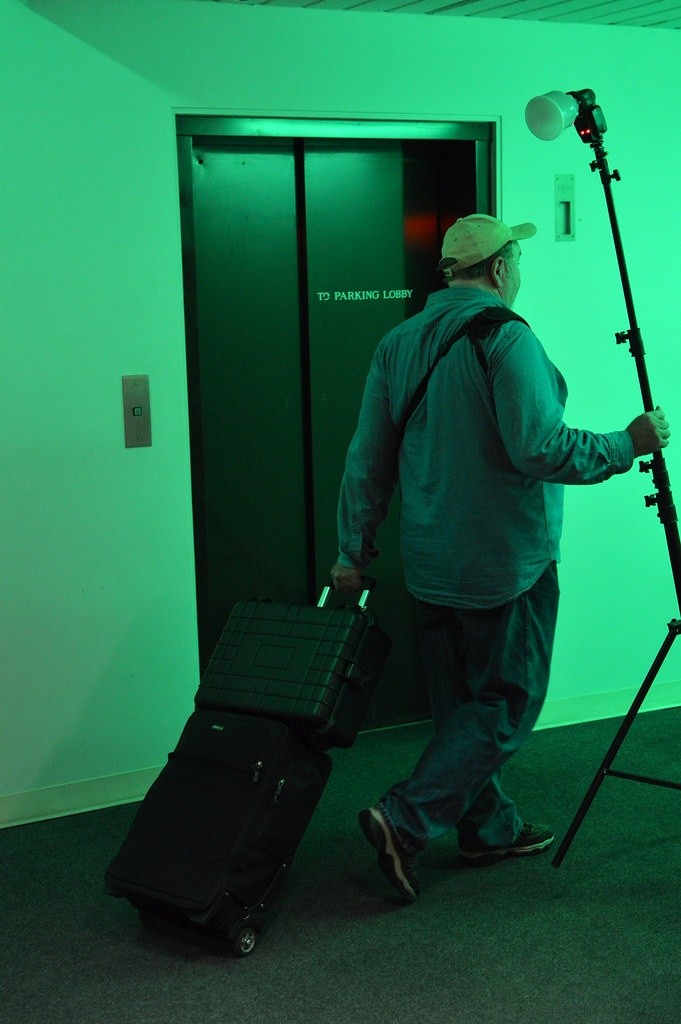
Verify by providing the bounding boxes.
[101,575,376,958]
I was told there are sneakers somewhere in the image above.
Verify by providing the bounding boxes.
[356,805,422,903]
[456,824,555,866]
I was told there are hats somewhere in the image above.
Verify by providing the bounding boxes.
[439,214,537,275]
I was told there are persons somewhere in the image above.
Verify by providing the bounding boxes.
[331,211,673,904]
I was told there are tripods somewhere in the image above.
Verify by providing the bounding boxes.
[547,89,681,868]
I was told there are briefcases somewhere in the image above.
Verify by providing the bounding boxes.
[192,598,391,751]
